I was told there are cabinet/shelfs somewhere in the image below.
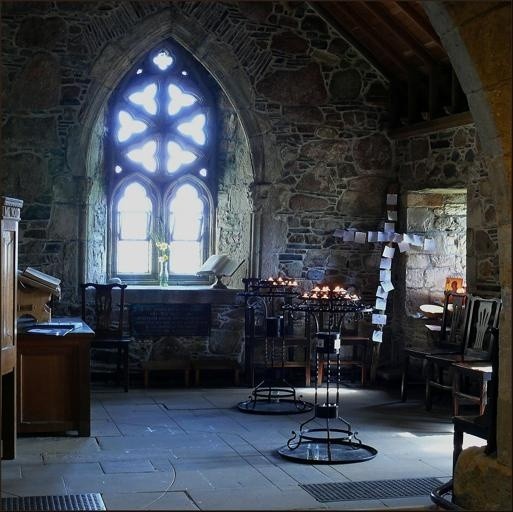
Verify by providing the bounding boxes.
[0,197,22,458]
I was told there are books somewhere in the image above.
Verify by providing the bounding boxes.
[33,321,82,329]
[194,254,244,276]
[21,267,61,289]
[26,328,72,337]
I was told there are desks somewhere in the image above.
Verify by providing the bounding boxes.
[14,316,93,438]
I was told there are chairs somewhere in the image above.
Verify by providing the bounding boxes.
[244,280,369,388]
[77,281,130,393]
[398,289,502,479]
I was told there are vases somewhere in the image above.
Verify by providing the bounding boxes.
[159,262,168,287]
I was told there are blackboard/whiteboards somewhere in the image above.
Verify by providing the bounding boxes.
[128,302,211,337]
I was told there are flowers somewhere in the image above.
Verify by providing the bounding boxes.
[149,221,176,286]
